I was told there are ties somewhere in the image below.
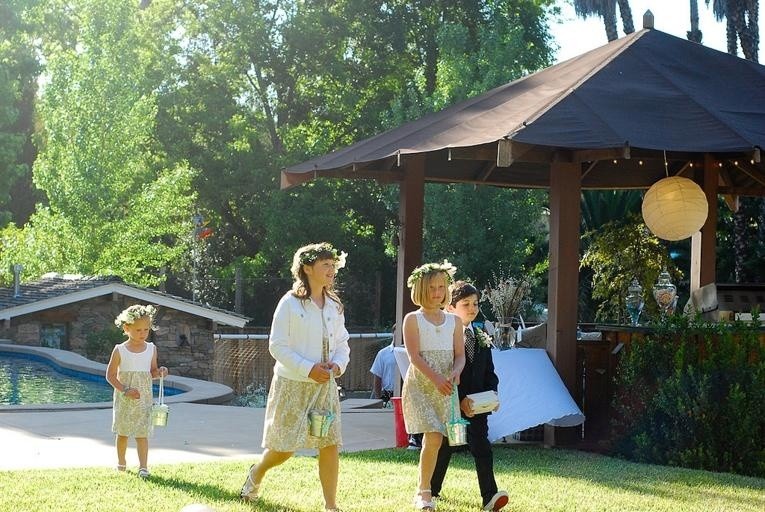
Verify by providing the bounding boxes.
[464,328,476,364]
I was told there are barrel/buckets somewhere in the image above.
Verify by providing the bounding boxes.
[390,395,410,448]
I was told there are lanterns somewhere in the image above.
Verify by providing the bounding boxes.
[642,176,710,242]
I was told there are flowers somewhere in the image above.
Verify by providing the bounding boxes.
[406,259,471,292]
[113,302,157,328]
[471,260,543,347]
[295,241,348,275]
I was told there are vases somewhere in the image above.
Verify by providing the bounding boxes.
[489,319,516,348]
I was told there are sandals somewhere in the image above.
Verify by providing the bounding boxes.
[414,488,436,511]
[137,468,150,479]
[240,464,261,505]
[118,461,126,472]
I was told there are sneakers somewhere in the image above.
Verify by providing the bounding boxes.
[483,490,509,512]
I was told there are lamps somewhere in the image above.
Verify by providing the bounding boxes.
[638,153,710,243]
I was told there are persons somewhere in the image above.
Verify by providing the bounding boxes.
[429,279,510,511]
[104,306,169,480]
[241,242,352,512]
[399,260,467,510]
[367,320,404,409]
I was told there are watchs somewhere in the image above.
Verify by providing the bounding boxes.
[121,384,130,396]
